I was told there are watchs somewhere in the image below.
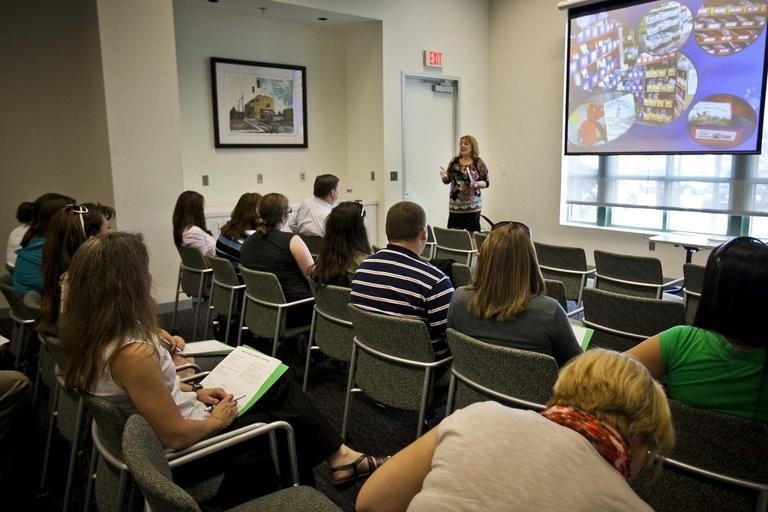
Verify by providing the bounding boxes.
[192,383,203,392]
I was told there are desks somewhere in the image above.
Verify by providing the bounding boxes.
[650,232,768,299]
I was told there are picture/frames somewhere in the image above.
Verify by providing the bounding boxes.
[209,57,312,150]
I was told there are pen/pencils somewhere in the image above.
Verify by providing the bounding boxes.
[203,395,246,411]
[159,335,186,356]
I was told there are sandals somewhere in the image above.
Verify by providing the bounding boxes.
[332,452,394,492]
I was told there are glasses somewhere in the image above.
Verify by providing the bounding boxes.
[338,201,365,217]
[67,204,88,241]
[714,236,767,269]
[488,221,532,242]
[285,206,292,213]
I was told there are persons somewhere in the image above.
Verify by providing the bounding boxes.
[212,193,263,300]
[438,136,489,232]
[288,173,340,240]
[59,231,391,512]
[1,369,33,469]
[172,190,215,266]
[620,236,768,421]
[240,193,315,329]
[307,201,375,290]
[5,202,36,274]
[36,202,195,393]
[350,201,455,388]
[447,220,584,367]
[13,193,77,316]
[355,349,675,512]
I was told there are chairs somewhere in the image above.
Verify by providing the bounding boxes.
[236,263,316,360]
[2,275,40,388]
[440,328,558,431]
[302,280,359,395]
[537,278,586,334]
[417,258,472,293]
[54,372,214,509]
[296,231,325,263]
[31,332,204,455]
[580,286,687,360]
[416,225,435,259]
[437,227,478,270]
[626,397,767,511]
[532,240,596,320]
[593,248,687,308]
[344,303,453,445]
[204,251,246,346]
[473,230,485,278]
[81,396,284,512]
[171,242,211,339]
[682,264,703,328]
[119,414,345,511]
